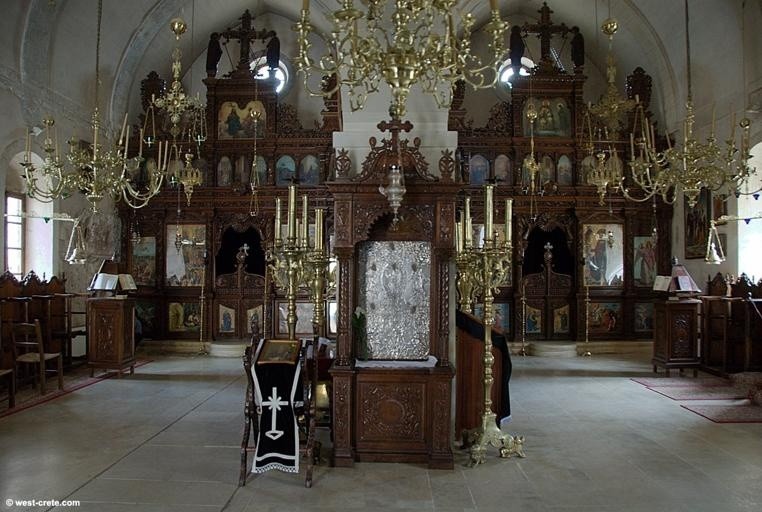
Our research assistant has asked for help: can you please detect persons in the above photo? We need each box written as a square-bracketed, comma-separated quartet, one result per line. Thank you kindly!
[557, 102, 570, 132]
[527, 104, 538, 134]
[185, 311, 200, 327]
[639, 240, 656, 285]
[250, 310, 259, 336]
[225, 106, 256, 135]
[223, 310, 231, 331]
[603, 309, 617, 332]
[494, 312, 504, 331]
[558, 309, 567, 330]
[526, 311, 537, 331]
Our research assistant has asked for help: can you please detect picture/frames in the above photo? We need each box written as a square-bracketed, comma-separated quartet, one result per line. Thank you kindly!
[714, 233, 727, 256]
[712, 194, 727, 226]
[684, 185, 711, 259]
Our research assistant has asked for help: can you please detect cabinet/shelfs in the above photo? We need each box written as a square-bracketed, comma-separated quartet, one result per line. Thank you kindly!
[649, 296, 702, 378]
[83, 296, 137, 382]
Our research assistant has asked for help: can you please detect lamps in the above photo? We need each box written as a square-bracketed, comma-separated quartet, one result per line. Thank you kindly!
[584, 121, 620, 204]
[288, 0, 514, 120]
[17, 0, 174, 217]
[614, 1, 756, 208]
[580, 1, 644, 159]
[143, 5, 213, 147]
[172, 129, 206, 208]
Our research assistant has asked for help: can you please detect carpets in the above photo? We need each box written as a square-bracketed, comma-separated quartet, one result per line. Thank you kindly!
[679, 401, 761, 425]
[644, 384, 747, 400]
[1, 358, 156, 418]
[628, 371, 730, 386]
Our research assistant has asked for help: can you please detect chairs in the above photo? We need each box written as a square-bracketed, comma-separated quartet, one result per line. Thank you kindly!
[9, 319, 66, 396]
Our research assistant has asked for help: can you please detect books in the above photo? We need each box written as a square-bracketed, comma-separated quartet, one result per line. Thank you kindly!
[93, 272, 137, 291]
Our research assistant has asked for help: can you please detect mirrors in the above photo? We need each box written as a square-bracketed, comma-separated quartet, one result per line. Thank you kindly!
[580, 222, 624, 290]
[163, 219, 207, 289]
